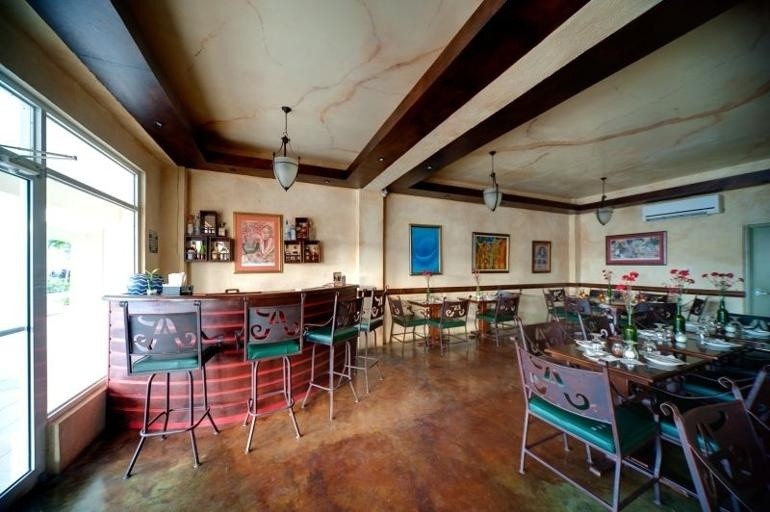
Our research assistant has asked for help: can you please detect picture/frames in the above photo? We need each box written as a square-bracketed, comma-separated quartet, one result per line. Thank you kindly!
[472, 232, 508, 273]
[531, 241, 551, 273]
[233, 211, 282, 273]
[606, 231, 666, 265]
[409, 224, 442, 276]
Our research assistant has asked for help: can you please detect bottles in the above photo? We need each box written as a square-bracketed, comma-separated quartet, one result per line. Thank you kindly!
[285, 246, 299, 262]
[333, 272, 345, 286]
[187, 239, 205, 260]
[624, 313, 637, 342]
[611, 321, 725, 360]
[305, 247, 318, 262]
[283, 220, 306, 241]
[187, 215, 226, 237]
[476, 286, 480, 294]
[212, 242, 229, 261]
[717, 300, 729, 324]
[674, 304, 685, 332]
[426, 288, 430, 301]
[607, 284, 611, 296]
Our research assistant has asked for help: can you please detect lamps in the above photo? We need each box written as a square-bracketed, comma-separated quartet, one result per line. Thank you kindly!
[483, 151, 502, 211]
[272, 106, 300, 190]
[595, 176, 615, 225]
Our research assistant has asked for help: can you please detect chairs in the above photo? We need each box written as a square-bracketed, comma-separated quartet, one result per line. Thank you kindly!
[301, 290, 364, 420]
[336, 285, 388, 394]
[243, 293, 307, 455]
[119, 301, 221, 479]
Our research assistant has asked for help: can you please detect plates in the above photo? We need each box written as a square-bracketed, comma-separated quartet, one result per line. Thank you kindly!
[643, 351, 690, 367]
[741, 329, 770, 336]
[702, 339, 743, 348]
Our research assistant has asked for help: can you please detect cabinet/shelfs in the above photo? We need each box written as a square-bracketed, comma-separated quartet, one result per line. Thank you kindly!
[284, 218, 321, 263]
[184, 235, 209, 263]
[209, 236, 233, 262]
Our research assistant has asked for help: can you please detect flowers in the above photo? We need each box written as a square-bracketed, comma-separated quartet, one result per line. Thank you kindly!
[703, 272, 745, 298]
[602, 269, 614, 285]
[421, 272, 434, 292]
[662, 268, 694, 303]
[615, 271, 646, 313]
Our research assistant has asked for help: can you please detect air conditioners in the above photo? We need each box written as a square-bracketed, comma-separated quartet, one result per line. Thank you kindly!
[641, 194, 725, 222]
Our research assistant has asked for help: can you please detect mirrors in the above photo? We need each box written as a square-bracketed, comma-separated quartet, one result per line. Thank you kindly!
[200, 211, 218, 236]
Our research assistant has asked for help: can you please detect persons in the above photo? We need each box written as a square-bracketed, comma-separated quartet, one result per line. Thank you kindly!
[242, 225, 275, 257]
